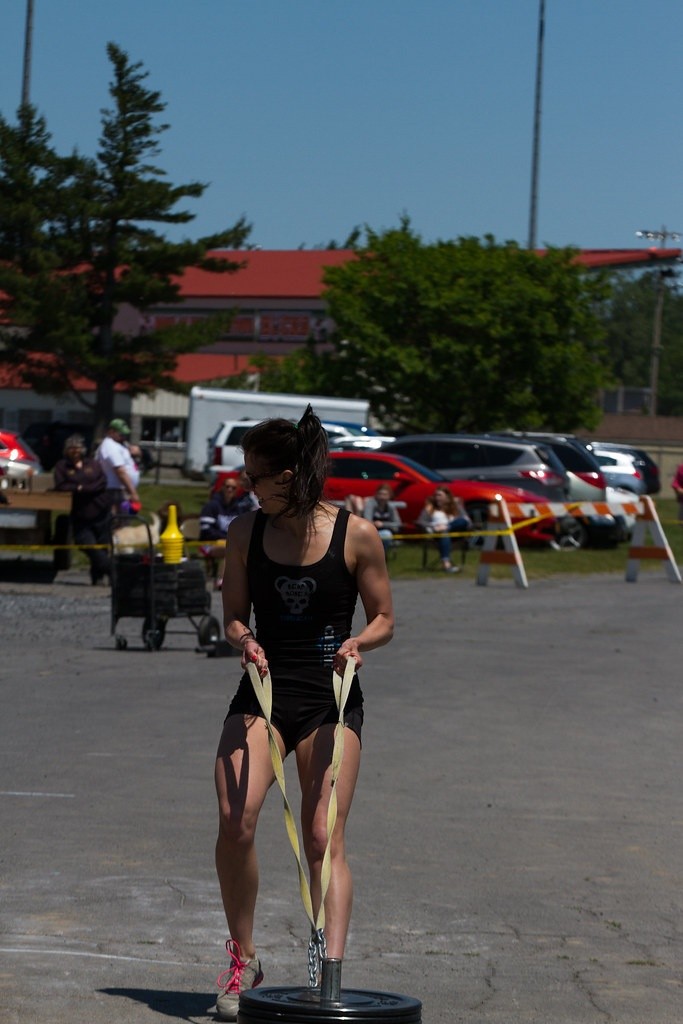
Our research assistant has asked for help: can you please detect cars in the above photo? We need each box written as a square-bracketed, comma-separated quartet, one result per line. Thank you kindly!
[185, 385, 662, 554]
[0, 429, 43, 498]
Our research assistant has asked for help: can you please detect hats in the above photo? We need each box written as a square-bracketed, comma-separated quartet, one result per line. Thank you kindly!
[109, 419, 133, 436]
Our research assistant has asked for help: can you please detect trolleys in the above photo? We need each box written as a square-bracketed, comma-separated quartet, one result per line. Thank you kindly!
[105, 509, 221, 653]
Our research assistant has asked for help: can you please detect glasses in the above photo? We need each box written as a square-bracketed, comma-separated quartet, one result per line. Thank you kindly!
[224, 484, 238, 490]
[244, 470, 277, 486]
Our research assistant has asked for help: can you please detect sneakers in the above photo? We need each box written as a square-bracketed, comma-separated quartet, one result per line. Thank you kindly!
[218, 955, 261, 1018]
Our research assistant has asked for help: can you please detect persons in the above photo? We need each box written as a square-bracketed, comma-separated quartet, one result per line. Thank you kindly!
[96, 419, 141, 528]
[212, 403, 394, 1021]
[671, 460, 683, 516]
[363, 483, 401, 563]
[415, 484, 473, 574]
[234, 467, 262, 513]
[54, 434, 115, 584]
[198, 477, 239, 590]
